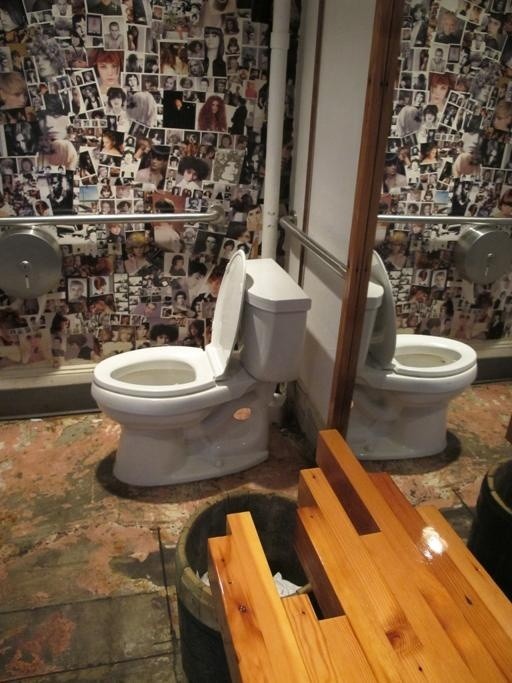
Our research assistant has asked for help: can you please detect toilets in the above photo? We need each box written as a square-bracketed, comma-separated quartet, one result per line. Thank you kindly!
[91, 250, 312, 486]
[349, 251, 479, 462]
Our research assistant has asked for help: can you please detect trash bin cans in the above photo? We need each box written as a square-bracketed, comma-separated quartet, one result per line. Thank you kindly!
[175, 489, 315, 683]
[465, 458, 512, 603]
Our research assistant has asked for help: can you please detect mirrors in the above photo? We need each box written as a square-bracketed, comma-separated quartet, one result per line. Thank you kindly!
[333, 2, 512, 610]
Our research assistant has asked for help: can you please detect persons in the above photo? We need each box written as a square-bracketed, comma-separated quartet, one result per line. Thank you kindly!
[0, 206, 286, 362]
[376, 223, 512, 342]
[0, 1, 294, 218]
[378, 1, 512, 218]
